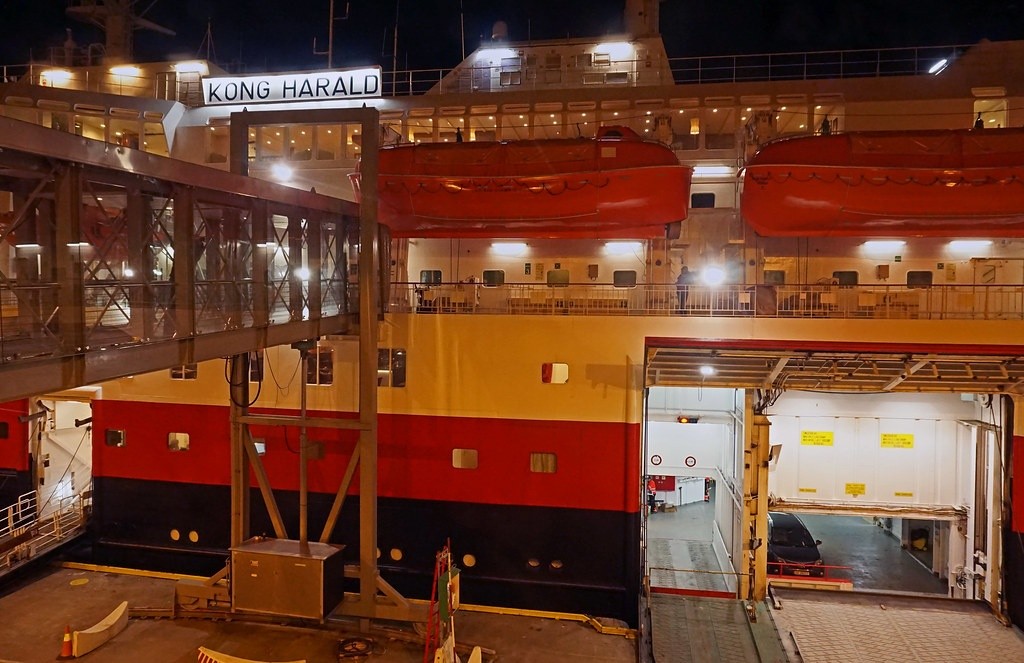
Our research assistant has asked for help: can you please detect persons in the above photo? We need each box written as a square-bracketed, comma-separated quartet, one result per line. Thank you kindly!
[648, 476, 656, 514]
[675, 266, 692, 314]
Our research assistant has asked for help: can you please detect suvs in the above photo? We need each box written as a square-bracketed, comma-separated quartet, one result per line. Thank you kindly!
[767, 511, 826, 577]
